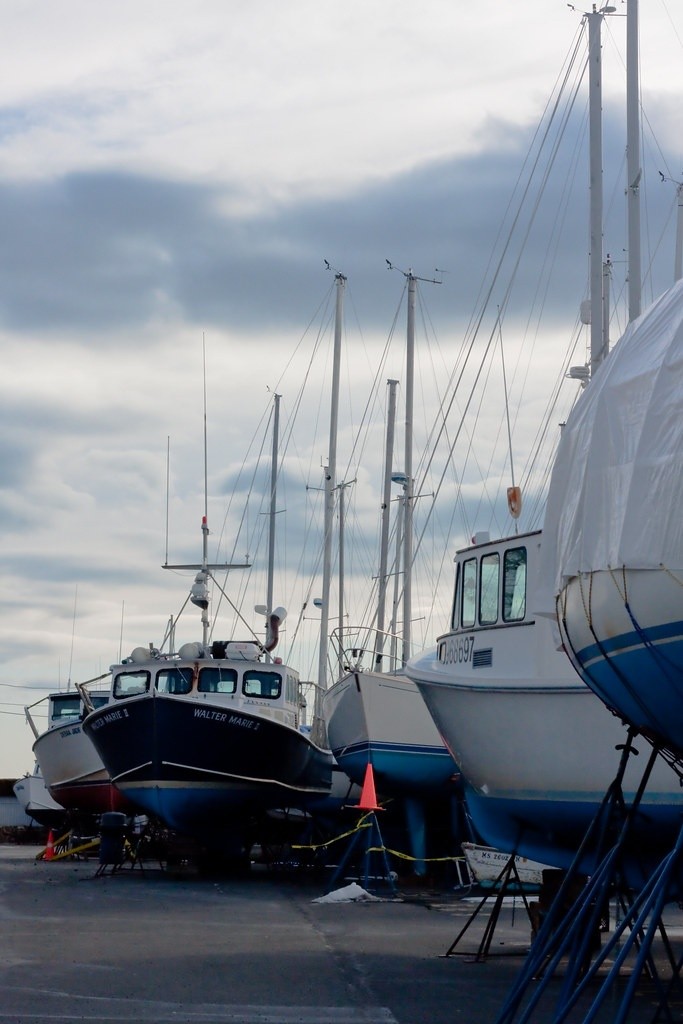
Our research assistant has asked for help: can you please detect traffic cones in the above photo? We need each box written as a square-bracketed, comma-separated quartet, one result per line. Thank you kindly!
[43, 831, 56, 859]
[353, 762, 384, 811]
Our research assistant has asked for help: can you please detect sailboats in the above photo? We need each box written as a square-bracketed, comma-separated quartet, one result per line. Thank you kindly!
[8, 1, 683, 886]
